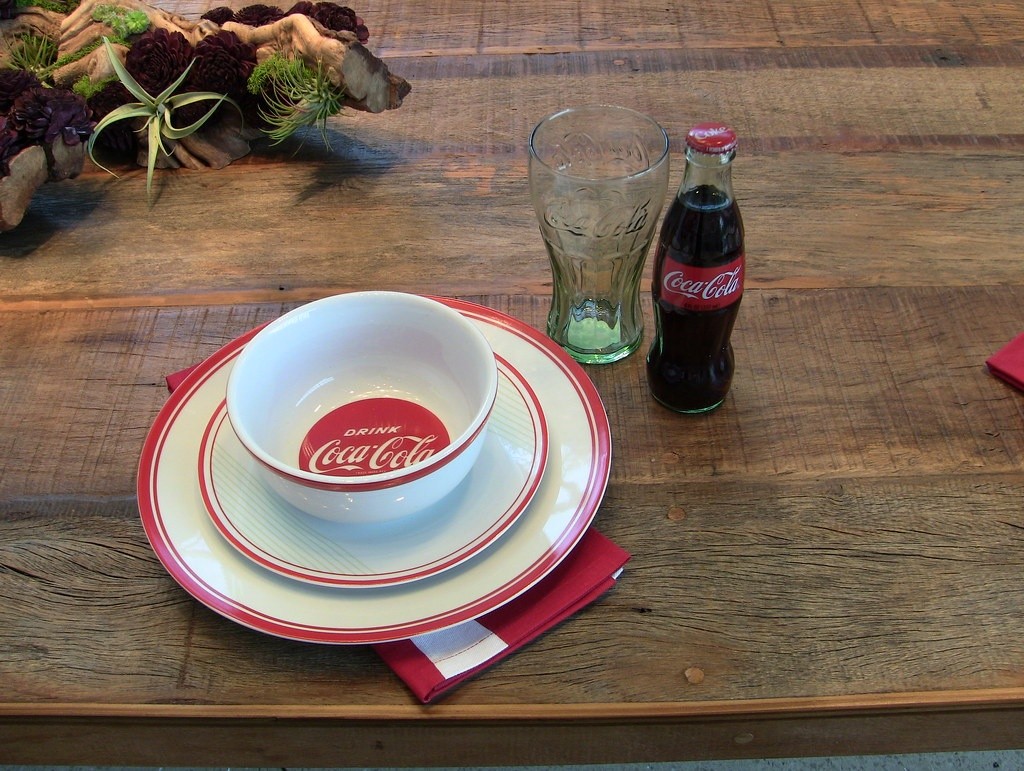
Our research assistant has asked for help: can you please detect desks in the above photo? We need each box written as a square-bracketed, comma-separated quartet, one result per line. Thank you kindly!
[1, 0, 1023, 771]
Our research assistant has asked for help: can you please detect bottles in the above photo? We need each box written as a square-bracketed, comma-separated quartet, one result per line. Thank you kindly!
[647, 124, 745, 416]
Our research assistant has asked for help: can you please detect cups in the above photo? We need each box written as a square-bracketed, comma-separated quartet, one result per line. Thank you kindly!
[530, 103, 669, 365]
[226, 290, 499, 526]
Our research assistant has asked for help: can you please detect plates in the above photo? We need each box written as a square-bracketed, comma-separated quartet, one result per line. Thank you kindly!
[199, 353, 549, 589]
[137, 295, 611, 644]
